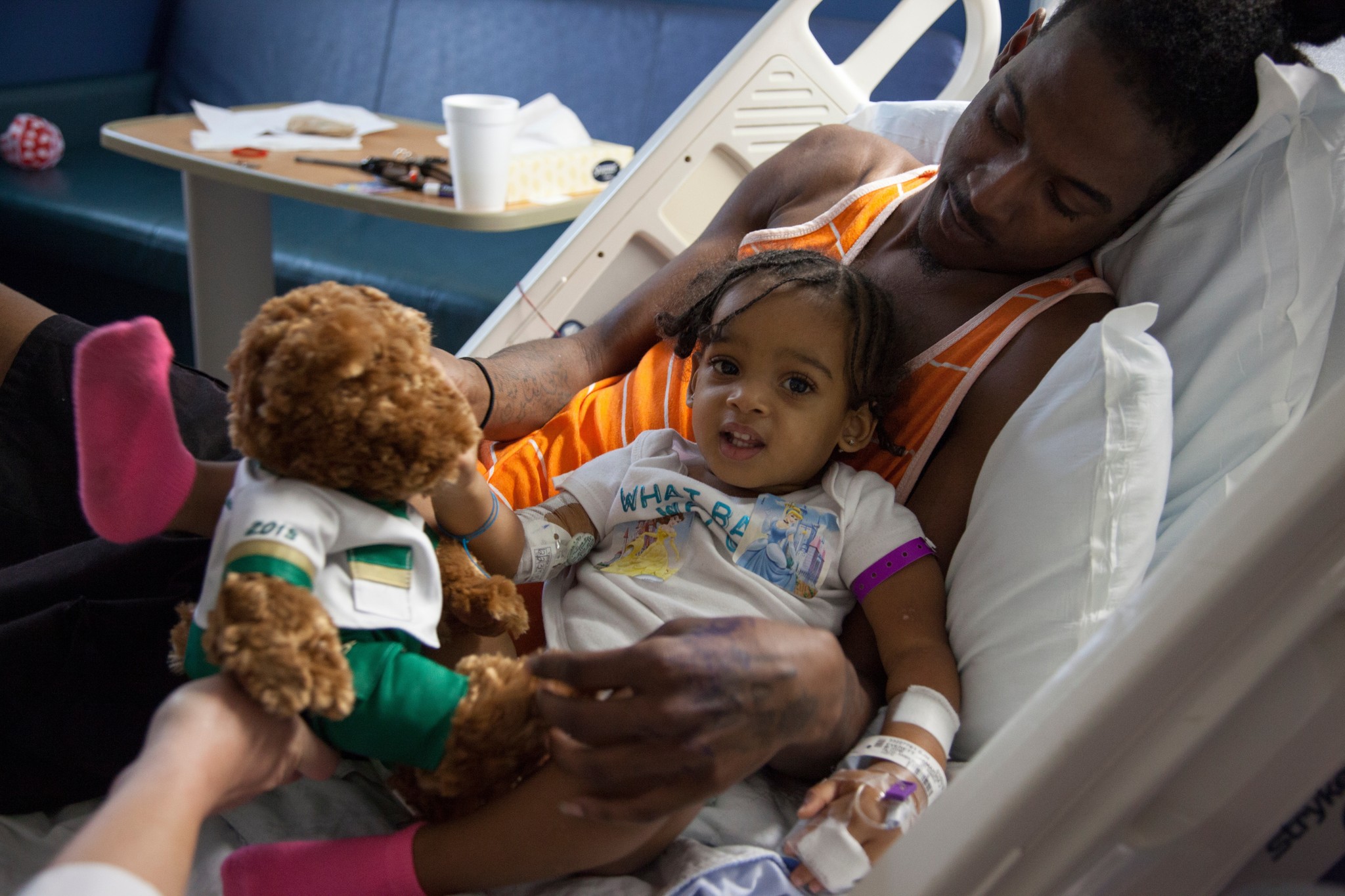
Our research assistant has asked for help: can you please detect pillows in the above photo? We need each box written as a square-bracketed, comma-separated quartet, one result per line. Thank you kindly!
[947, 301, 1172, 768]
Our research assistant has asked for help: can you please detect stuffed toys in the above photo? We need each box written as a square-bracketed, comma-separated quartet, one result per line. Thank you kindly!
[168, 277, 571, 827]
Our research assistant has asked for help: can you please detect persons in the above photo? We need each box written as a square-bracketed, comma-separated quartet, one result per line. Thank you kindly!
[0, 0, 1259, 896]
[72, 250, 966, 896]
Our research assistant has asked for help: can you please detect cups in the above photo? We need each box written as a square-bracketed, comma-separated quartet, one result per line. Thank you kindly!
[441, 87, 519, 219]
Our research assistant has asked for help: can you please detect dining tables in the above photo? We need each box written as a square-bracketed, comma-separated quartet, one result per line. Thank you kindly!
[97, 93, 638, 389]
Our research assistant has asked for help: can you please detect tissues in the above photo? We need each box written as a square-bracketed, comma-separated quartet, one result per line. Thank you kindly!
[436, 93, 635, 202]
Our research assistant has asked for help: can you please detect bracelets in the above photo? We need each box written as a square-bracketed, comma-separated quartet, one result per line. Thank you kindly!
[849, 535, 938, 604]
[461, 493, 499, 577]
[844, 731, 949, 806]
[460, 356, 495, 433]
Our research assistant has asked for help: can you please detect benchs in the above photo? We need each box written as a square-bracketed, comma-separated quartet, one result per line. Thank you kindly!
[4, 116, 582, 348]
